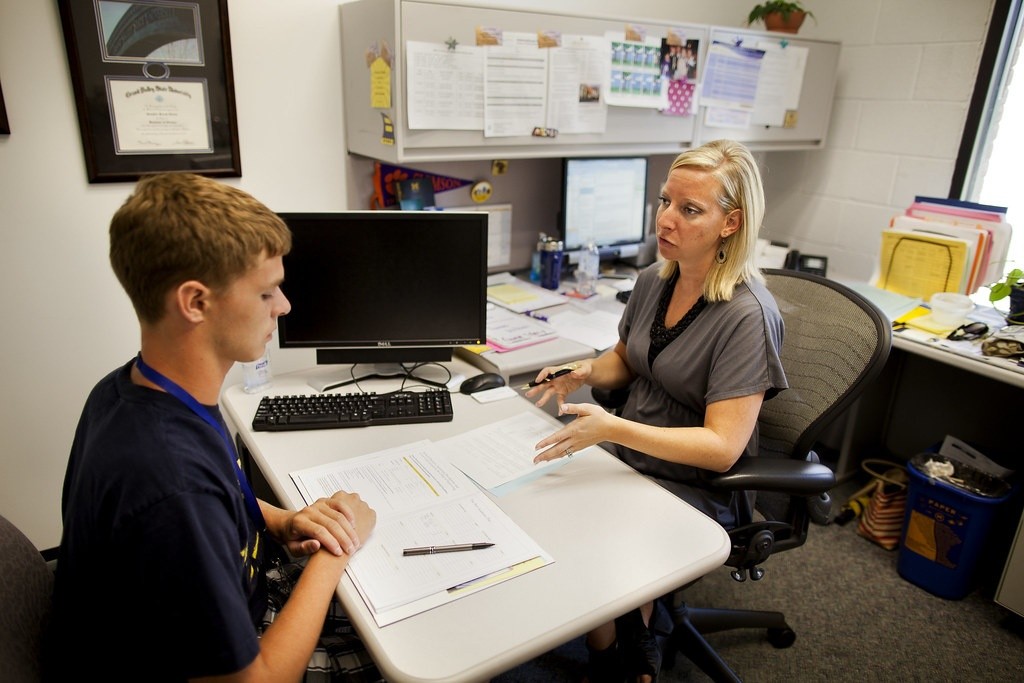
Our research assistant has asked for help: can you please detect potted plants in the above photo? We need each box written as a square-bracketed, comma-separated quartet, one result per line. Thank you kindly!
[743, 0, 815, 35]
[982, 258, 1023, 329]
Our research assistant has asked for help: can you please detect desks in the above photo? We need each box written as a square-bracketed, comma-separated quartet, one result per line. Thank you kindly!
[218, 238, 1024, 683]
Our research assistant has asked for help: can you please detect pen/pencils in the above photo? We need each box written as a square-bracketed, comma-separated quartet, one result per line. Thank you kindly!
[403, 542, 495, 556]
[520, 365, 578, 390]
[525, 310, 547, 321]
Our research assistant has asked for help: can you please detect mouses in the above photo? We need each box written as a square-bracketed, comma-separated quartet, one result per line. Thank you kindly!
[459, 373, 505, 395]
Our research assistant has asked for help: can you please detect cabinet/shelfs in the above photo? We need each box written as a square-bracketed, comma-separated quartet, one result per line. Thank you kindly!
[338, 0, 840, 177]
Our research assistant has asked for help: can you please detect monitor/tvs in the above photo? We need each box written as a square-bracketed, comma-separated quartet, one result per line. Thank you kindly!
[274, 212, 488, 392]
[559, 157, 649, 281]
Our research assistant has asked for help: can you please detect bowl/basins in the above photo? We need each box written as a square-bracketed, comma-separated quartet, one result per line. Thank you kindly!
[930, 293, 975, 325]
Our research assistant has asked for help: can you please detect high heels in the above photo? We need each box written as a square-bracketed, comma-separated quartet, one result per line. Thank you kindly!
[584, 598, 674, 683]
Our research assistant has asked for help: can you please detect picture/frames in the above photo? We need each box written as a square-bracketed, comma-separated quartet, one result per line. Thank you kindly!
[55, 0, 245, 182]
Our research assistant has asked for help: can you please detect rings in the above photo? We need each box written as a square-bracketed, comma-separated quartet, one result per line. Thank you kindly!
[566, 449, 572, 458]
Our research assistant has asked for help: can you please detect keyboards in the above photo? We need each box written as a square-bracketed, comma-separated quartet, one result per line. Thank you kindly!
[252, 388, 454, 432]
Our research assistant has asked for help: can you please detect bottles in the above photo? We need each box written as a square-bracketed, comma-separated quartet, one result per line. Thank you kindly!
[576, 236, 599, 295]
[529, 240, 564, 291]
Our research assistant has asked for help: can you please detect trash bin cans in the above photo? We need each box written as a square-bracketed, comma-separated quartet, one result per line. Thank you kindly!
[895, 452, 1004, 603]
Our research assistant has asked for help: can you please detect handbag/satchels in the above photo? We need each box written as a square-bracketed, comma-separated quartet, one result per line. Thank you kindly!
[843, 460, 910, 551]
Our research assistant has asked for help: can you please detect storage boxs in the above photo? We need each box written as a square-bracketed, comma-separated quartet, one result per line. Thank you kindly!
[899, 435, 1022, 605]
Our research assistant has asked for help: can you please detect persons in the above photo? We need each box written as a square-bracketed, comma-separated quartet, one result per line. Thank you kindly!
[660, 46, 696, 78]
[36, 172, 376, 683]
[524, 139, 789, 683]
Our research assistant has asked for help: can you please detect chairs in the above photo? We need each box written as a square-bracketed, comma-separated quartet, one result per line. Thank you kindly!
[595, 264, 890, 682]
[0, 516, 57, 683]
